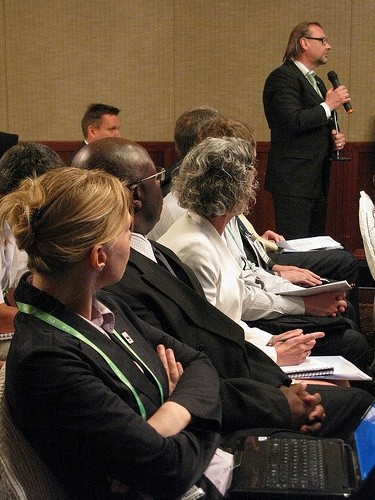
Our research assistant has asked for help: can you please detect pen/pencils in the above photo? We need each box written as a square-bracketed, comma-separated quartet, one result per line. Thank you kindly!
[277, 339, 288, 342]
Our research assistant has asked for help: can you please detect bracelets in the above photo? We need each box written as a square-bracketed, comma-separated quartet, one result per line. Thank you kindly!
[277, 271, 281, 277]
[268, 337, 273, 346]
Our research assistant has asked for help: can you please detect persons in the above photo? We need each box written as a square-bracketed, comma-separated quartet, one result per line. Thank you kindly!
[0, 103, 372, 500]
[263, 22, 351, 241]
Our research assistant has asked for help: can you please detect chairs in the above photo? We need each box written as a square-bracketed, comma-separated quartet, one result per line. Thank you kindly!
[0, 360, 71, 500]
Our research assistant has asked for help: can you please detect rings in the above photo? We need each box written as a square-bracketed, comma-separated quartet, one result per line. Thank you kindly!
[301, 275, 306, 279]
[307, 352, 310, 356]
[339, 143, 341, 147]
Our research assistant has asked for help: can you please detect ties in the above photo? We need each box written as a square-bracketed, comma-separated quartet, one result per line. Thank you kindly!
[306, 74, 323, 99]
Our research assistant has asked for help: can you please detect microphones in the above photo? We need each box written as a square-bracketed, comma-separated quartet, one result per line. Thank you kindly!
[327, 71, 353, 114]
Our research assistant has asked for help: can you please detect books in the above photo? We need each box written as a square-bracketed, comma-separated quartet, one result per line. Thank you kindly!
[280, 358, 335, 379]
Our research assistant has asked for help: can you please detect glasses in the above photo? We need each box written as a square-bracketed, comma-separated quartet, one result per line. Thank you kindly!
[135, 166, 167, 184]
[305, 36, 328, 45]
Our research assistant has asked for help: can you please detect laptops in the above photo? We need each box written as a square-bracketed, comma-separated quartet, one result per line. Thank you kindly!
[226, 399, 375, 500]
